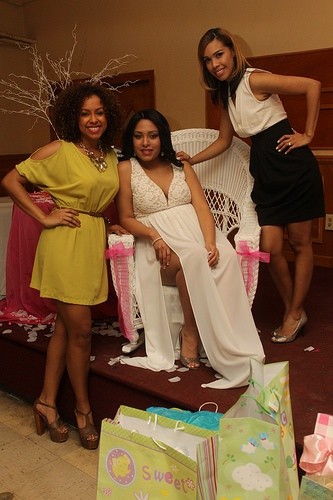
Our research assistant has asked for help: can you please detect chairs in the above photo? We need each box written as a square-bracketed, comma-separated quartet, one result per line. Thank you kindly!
[108, 128, 261, 352]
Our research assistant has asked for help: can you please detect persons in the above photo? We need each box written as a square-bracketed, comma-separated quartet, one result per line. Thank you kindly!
[176, 28, 325, 342]
[1, 84, 119, 449]
[118, 109, 266, 388]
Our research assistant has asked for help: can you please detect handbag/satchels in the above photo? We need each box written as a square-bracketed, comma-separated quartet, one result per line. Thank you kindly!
[95, 357, 333, 500]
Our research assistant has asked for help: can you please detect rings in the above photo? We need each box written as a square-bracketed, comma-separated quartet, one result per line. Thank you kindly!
[289, 142, 291, 145]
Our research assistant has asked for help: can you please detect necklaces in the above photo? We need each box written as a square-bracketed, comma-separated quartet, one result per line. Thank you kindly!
[80, 141, 107, 173]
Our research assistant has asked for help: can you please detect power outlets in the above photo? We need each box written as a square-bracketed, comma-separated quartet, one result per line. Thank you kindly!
[325, 214, 333, 230]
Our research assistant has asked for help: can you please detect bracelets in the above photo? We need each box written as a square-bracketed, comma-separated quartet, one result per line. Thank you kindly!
[152, 237, 161, 246]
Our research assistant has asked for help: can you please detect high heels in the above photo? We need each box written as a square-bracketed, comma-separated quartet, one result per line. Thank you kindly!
[74, 406, 100, 448]
[272, 311, 308, 342]
[32, 398, 69, 442]
[178, 330, 200, 369]
